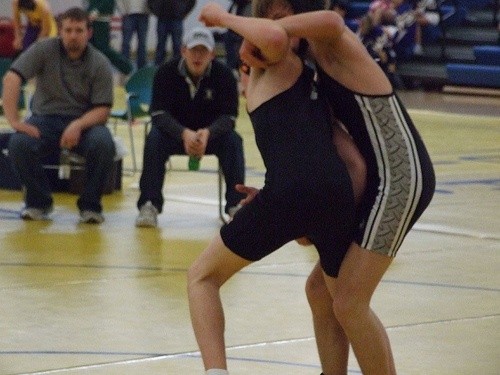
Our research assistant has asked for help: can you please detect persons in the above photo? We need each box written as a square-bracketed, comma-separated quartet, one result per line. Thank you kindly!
[114, 0, 148, 72]
[329, 0, 358, 32]
[12, 0, 58, 51]
[137, 27, 245, 229]
[147, 0, 196, 68]
[250, 0, 436, 375]
[186, 2, 366, 375]
[3, 8, 114, 223]
[353, 0, 457, 86]
[86, 0, 115, 55]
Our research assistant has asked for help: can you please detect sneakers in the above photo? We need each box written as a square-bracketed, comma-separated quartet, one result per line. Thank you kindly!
[79, 210, 106, 223]
[135, 200, 160, 229]
[20, 202, 55, 220]
[220, 206, 235, 225]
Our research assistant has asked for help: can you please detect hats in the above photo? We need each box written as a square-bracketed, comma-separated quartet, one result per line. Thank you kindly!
[182, 27, 215, 51]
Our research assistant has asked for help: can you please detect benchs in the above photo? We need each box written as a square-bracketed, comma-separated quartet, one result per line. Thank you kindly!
[349, 0, 500, 89]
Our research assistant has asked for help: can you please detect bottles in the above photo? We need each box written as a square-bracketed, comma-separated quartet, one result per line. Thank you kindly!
[189, 156, 199, 171]
[58, 142, 71, 180]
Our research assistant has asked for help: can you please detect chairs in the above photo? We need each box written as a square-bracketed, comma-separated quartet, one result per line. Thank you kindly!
[0, 15, 228, 226]
